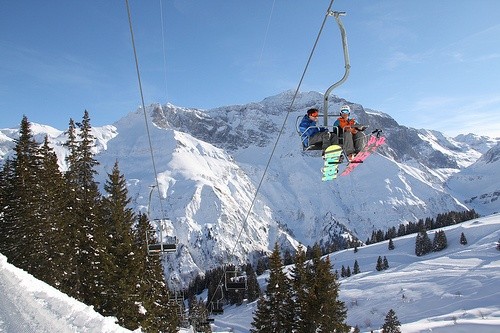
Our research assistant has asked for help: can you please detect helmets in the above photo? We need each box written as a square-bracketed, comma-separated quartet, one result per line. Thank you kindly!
[339, 105, 351, 115]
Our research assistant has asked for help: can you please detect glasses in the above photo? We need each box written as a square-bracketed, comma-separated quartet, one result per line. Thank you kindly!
[341, 110, 349, 114]
[310, 114, 318, 117]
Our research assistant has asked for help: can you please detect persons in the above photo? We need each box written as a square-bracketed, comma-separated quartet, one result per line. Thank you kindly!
[333, 106, 367, 161]
[299, 108, 344, 162]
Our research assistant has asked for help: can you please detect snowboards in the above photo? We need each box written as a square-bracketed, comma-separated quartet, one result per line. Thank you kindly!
[339, 137, 387, 176]
[321, 145, 343, 181]
